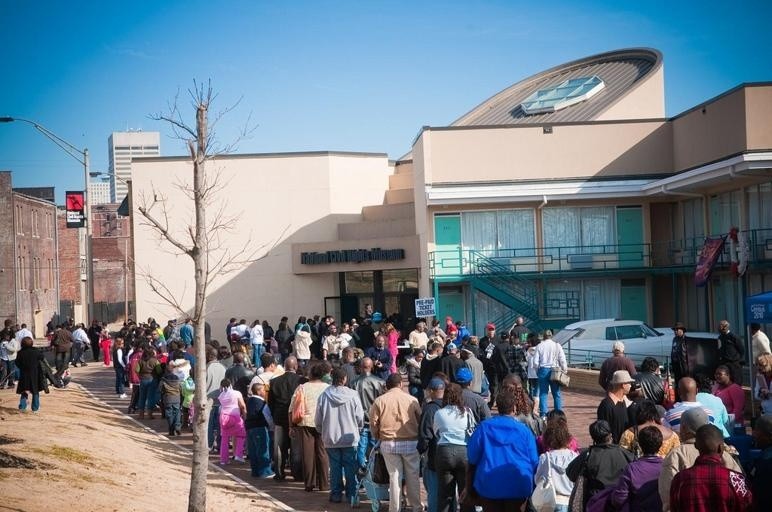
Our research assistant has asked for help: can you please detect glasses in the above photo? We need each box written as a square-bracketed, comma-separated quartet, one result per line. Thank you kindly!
[488, 329, 495, 332]
[713, 372, 727, 376]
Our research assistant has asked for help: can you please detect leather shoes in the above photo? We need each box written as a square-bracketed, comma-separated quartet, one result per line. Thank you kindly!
[305, 485, 316, 491]
[295, 477, 304, 482]
[273, 473, 287, 480]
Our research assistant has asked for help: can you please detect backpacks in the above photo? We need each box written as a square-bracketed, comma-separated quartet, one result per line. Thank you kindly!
[262, 383, 271, 402]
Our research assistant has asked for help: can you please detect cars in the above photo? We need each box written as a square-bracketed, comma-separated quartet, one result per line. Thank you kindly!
[542, 318, 724, 382]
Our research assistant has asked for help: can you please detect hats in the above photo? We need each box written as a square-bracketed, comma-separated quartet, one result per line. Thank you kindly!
[487, 324, 495, 329]
[454, 368, 473, 385]
[610, 370, 636, 386]
[612, 341, 624, 353]
[426, 378, 445, 390]
[446, 316, 453, 321]
[671, 322, 687, 334]
[501, 330, 510, 336]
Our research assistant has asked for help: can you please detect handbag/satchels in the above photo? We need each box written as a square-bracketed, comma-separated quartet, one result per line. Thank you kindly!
[629, 444, 640, 461]
[567, 475, 584, 512]
[468, 427, 478, 437]
[530, 475, 557, 512]
[52, 336, 61, 346]
[292, 393, 307, 425]
[550, 366, 571, 389]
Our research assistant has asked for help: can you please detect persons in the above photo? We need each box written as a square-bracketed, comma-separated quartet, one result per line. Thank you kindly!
[110, 303, 578, 512]
[1, 311, 112, 415]
[565, 319, 772, 512]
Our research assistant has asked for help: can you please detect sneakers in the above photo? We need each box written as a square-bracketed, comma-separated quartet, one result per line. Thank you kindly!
[234, 454, 246, 463]
[220, 459, 231, 465]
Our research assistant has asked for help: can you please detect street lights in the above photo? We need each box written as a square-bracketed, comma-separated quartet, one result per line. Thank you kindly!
[90, 170, 130, 330]
[2, 115, 97, 362]
